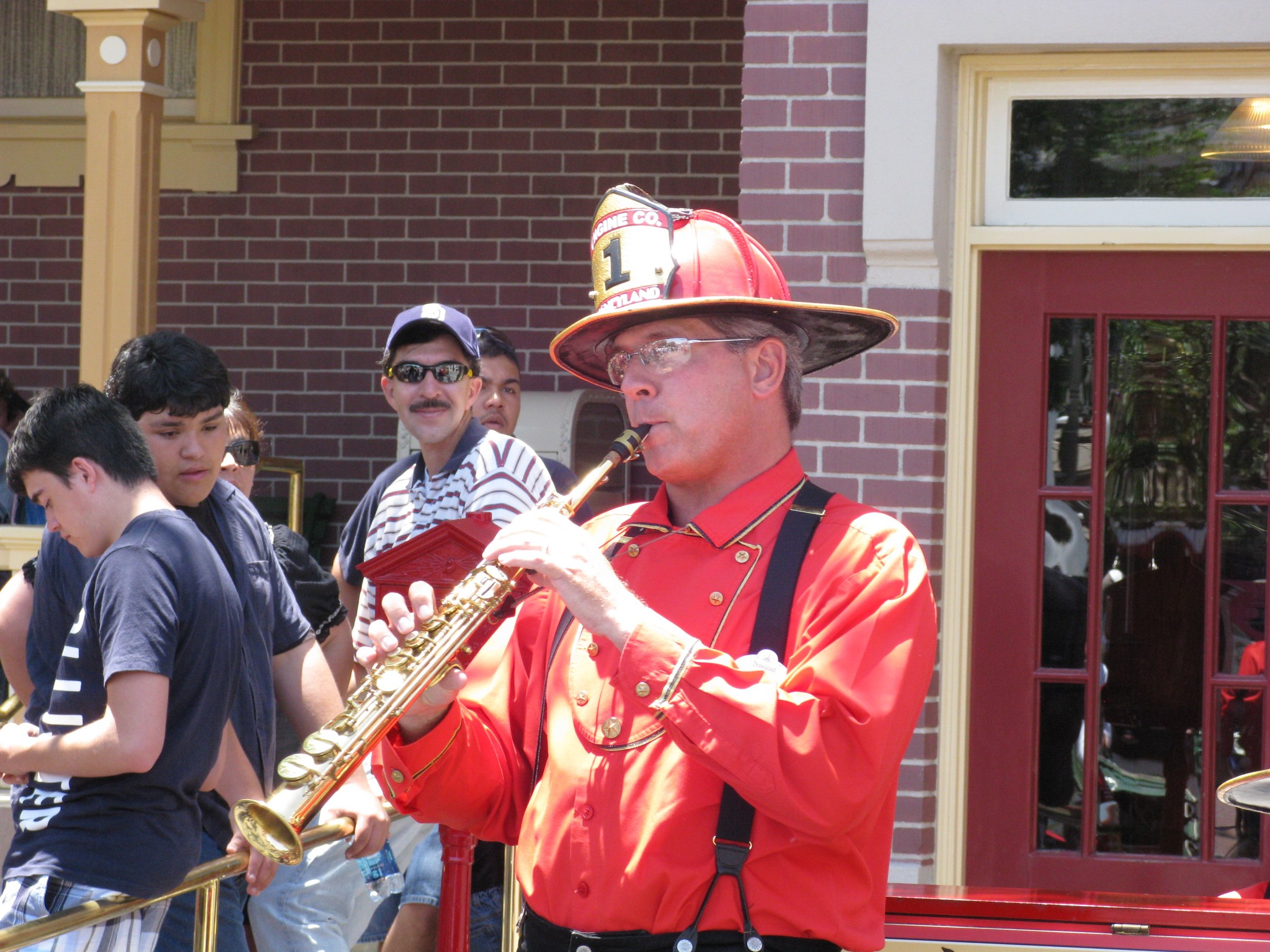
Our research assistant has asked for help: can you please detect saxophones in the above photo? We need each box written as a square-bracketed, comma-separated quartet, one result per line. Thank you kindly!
[234, 421, 655, 866]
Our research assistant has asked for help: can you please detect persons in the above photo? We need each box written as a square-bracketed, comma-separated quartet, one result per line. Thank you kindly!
[357, 184, 937, 952]
[0, 304, 591, 952]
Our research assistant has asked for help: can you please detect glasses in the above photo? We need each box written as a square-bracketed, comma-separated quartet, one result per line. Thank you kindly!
[222, 441, 260, 466]
[387, 362, 474, 383]
[606, 337, 755, 385]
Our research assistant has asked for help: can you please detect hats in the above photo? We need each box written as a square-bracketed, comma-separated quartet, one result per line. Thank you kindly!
[385, 302, 480, 357]
[549, 183, 899, 393]
